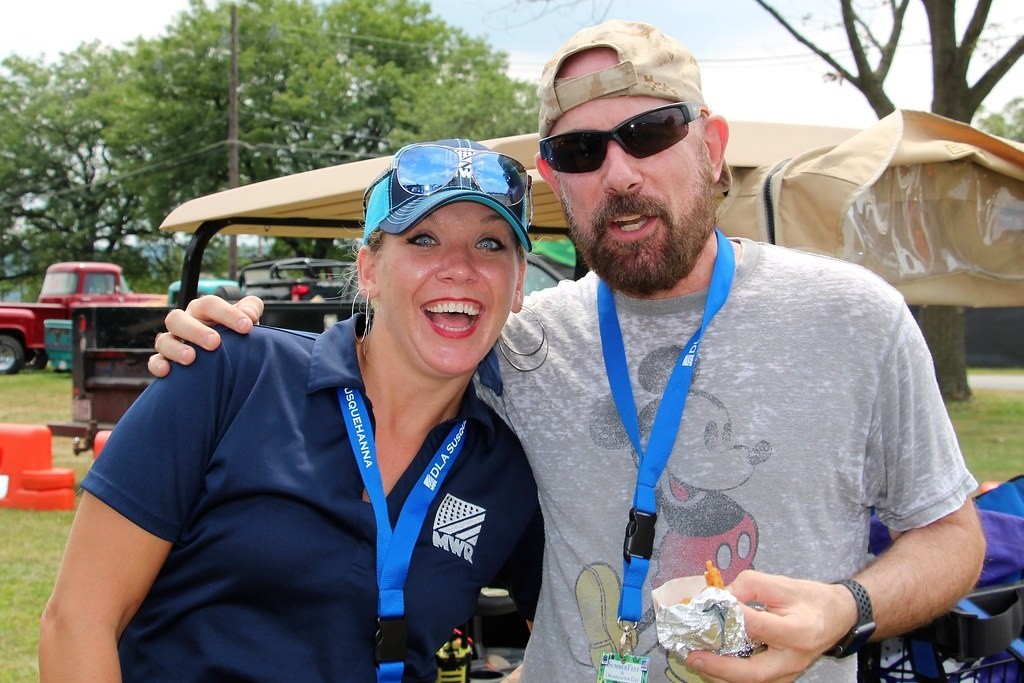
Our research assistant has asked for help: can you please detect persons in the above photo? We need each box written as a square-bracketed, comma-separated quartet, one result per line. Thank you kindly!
[147, 20, 987, 682]
[37, 136, 546, 683]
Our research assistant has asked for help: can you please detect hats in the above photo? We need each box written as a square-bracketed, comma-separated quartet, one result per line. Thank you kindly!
[362, 138, 532, 253]
[539, 20, 733, 192]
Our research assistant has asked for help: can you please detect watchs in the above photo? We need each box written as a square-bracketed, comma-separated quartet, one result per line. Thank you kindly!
[823, 579, 876, 658]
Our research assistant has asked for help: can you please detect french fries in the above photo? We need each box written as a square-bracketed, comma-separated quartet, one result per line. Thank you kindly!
[702, 560, 725, 588]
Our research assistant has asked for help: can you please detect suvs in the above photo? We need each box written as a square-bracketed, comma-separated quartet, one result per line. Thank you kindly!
[217, 256, 357, 303]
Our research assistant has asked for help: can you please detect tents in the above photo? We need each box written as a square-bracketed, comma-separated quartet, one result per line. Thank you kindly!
[156, 107, 1024, 305]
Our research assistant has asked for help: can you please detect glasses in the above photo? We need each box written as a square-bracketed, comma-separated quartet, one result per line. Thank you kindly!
[362, 143, 534, 227]
[539, 101, 703, 174]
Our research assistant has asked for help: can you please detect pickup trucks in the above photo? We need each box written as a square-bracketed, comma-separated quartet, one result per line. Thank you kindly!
[44, 280, 234, 373]
[0, 261, 169, 375]
